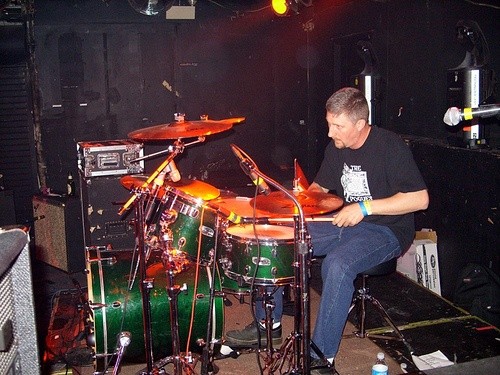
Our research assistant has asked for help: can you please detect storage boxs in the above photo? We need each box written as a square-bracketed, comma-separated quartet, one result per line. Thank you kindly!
[397, 229, 441, 297]
[76, 140, 144, 177]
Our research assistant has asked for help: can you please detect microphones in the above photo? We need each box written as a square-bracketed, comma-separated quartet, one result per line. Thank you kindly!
[169, 160, 181, 182]
[232, 145, 271, 196]
[443, 104, 500, 127]
[27, 215, 44, 223]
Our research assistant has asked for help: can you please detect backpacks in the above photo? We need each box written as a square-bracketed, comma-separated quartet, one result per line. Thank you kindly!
[454, 263, 500, 331]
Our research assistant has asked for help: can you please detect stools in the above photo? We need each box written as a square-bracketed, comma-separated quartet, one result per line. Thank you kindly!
[348, 258, 412, 354]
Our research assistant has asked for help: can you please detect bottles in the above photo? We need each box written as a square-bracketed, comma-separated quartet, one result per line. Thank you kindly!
[371, 352, 388, 375]
[67, 175, 74, 198]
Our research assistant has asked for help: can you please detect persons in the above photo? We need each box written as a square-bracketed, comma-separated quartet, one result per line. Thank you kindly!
[222, 87, 430, 375]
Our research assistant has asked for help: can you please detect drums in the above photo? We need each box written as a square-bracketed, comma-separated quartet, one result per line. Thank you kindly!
[84, 246, 225, 373]
[219, 224, 302, 287]
[143, 184, 223, 266]
[210, 195, 269, 243]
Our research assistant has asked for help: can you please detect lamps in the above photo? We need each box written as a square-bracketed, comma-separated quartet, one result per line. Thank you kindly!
[128, 0, 197, 20]
[271, 0, 312, 17]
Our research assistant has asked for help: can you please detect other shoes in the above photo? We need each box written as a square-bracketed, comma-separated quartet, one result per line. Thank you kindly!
[226, 321, 283, 346]
[310, 358, 336, 375]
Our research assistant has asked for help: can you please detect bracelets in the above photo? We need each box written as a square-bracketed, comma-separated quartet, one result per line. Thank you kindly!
[363, 200, 373, 215]
[358, 202, 367, 217]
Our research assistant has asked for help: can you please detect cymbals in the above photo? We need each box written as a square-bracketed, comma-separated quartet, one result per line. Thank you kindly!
[126, 119, 234, 140]
[248, 190, 344, 216]
[119, 174, 221, 201]
[220, 117, 246, 124]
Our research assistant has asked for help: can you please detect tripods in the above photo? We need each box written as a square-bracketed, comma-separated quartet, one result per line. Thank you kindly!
[230, 199, 339, 375]
[136, 210, 197, 375]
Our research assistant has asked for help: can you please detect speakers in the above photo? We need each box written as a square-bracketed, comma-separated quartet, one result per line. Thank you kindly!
[31, 194, 85, 274]
